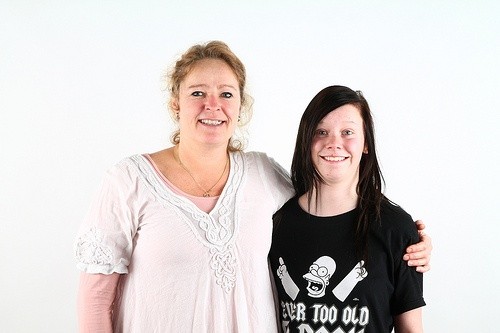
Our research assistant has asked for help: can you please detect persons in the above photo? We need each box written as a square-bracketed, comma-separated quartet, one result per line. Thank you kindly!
[267, 85, 427, 333]
[79, 40, 432, 333]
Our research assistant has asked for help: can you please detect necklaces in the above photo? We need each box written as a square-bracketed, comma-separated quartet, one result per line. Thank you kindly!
[176, 143, 230, 197]
